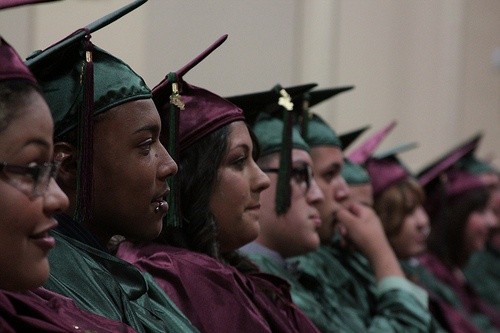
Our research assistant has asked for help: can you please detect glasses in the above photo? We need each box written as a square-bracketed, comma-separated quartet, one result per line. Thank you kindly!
[0, 160, 61, 195]
[262, 162, 314, 188]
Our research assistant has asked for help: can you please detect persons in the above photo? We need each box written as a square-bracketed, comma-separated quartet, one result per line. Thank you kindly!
[119, 35, 500, 333]
[0, 0, 132, 333]
[23, 0, 198, 333]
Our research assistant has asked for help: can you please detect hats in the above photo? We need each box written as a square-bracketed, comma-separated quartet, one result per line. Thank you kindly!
[225, 84, 317, 153]
[152, 33, 244, 148]
[0, 0, 65, 81]
[299, 84, 353, 147]
[415, 133, 500, 196]
[25, 0, 152, 143]
[341, 121, 395, 184]
[363, 142, 418, 193]
